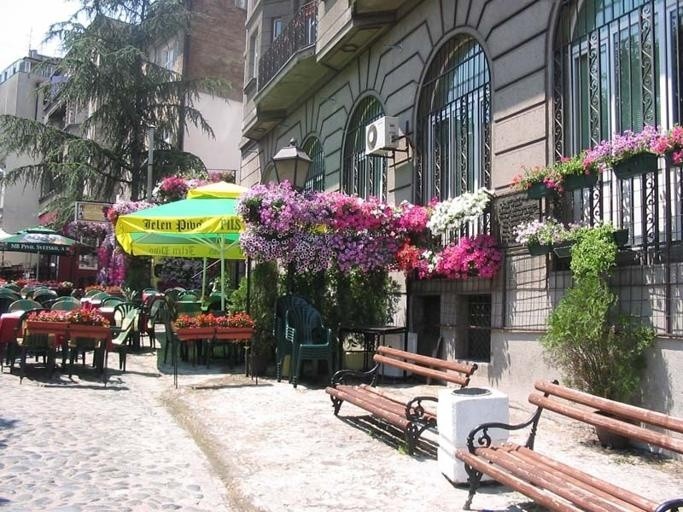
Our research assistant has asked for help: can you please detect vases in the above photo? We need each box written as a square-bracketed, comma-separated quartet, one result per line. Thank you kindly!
[550, 240, 577, 259]
[526, 243, 550, 257]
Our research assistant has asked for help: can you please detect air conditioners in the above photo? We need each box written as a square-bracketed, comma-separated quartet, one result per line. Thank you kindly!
[364, 115, 400, 156]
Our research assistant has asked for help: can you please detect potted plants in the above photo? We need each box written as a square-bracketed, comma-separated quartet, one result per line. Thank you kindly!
[539, 232, 655, 453]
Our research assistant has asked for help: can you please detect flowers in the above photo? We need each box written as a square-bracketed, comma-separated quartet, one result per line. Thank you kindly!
[510, 122, 682, 246]
[234, 174, 499, 279]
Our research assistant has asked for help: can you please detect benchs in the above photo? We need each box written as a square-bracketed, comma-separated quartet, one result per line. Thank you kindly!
[324, 344, 477, 456]
[454, 378, 682, 512]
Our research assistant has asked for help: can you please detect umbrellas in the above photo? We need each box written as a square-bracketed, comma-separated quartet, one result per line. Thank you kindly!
[0, 222, 98, 286]
[110, 179, 327, 312]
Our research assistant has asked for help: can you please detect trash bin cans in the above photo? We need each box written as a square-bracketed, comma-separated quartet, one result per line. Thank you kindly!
[437, 385, 509, 484]
